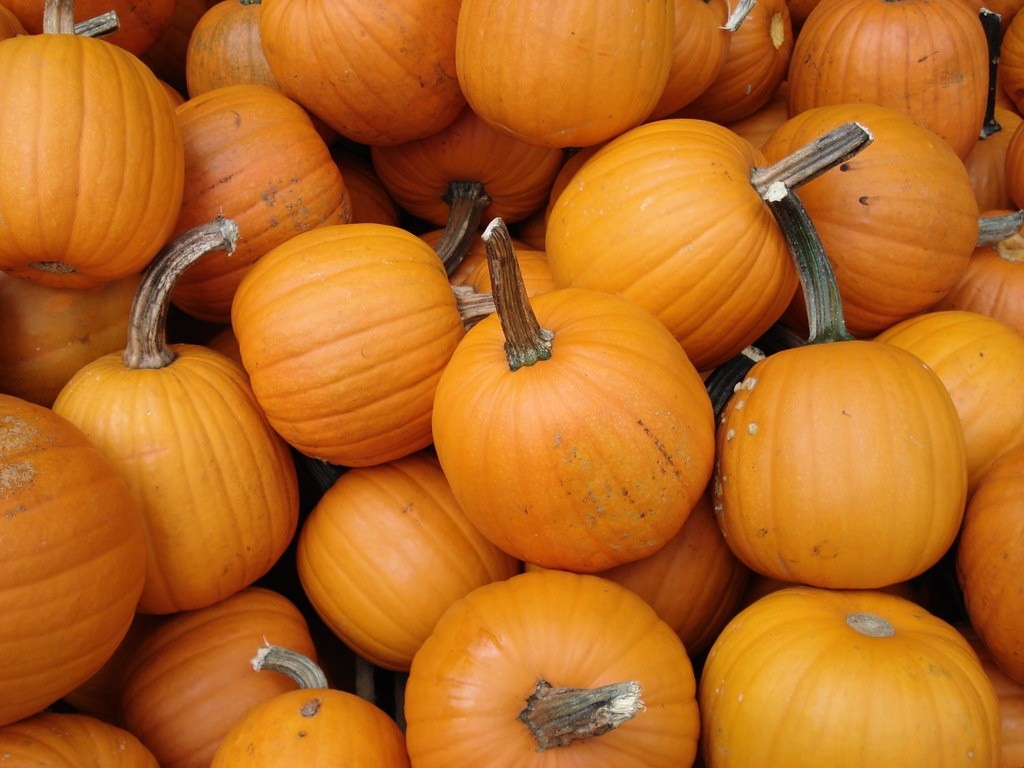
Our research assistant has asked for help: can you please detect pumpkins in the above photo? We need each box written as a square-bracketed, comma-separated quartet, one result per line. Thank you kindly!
[1, 0, 1024, 768]
